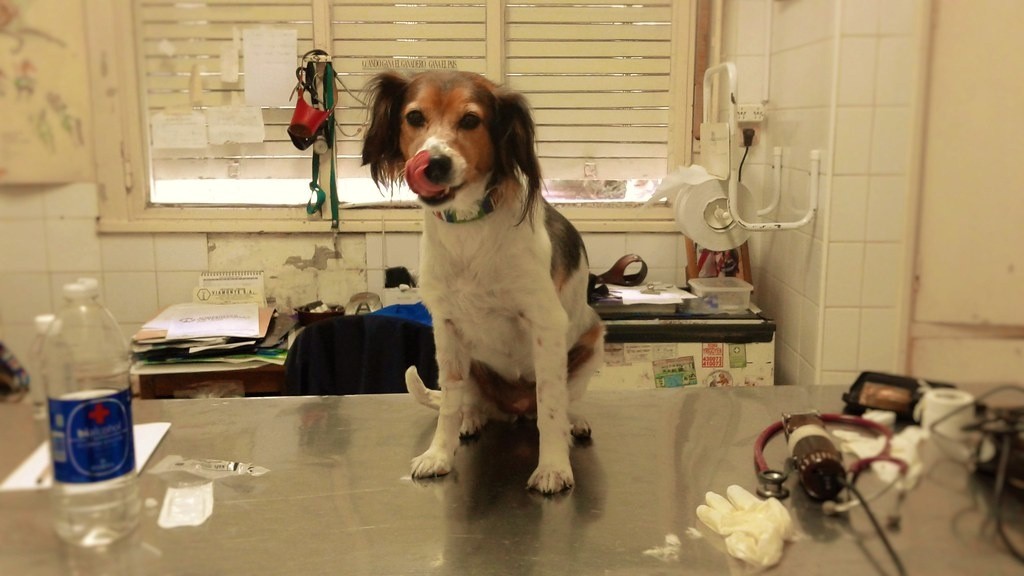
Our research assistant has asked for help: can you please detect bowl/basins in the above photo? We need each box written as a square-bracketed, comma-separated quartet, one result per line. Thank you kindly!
[688, 275, 755, 311]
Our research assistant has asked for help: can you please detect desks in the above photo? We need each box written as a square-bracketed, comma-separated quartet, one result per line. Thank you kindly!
[1, 378, 1024, 576]
[140, 365, 285, 399]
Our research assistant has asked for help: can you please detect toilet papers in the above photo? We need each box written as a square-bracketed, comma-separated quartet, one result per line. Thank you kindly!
[644, 165, 755, 251]
[914, 388, 974, 438]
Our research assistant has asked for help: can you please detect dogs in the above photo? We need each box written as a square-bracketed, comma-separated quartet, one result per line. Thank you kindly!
[360, 68, 608, 495]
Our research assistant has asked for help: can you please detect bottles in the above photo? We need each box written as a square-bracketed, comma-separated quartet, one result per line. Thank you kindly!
[25, 275, 144, 552]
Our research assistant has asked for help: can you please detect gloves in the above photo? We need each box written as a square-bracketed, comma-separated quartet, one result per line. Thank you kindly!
[697, 485, 793, 567]
[833, 427, 930, 491]
